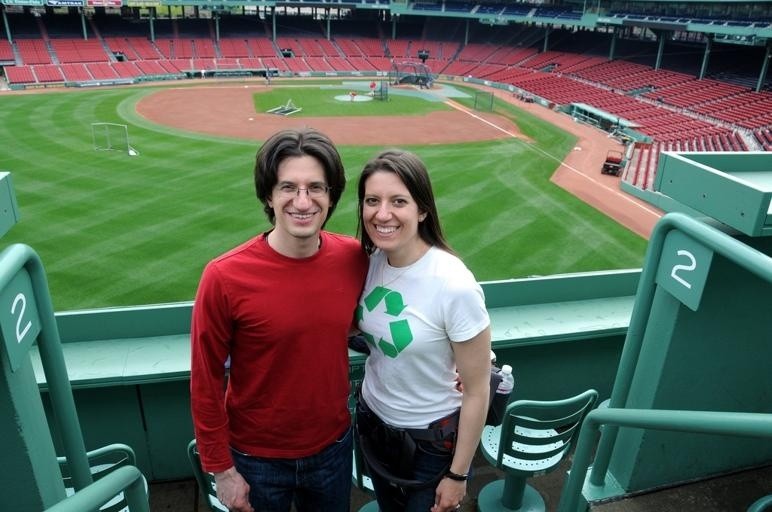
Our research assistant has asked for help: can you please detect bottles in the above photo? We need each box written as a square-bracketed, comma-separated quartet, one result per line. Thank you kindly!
[495, 364, 514, 394]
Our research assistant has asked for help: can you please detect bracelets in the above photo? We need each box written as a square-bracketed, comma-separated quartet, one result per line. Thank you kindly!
[445, 470, 469, 481]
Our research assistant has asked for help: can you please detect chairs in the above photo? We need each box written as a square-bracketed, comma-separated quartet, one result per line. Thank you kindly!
[352, 406, 381, 512]
[477, 389, 599, 512]
[56, 443, 149, 512]
[187, 438, 230, 512]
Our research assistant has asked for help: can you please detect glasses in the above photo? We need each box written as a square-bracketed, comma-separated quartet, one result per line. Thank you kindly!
[276, 182, 332, 199]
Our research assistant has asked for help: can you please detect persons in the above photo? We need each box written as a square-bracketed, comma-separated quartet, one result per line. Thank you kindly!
[349, 147, 492, 512]
[369, 80, 377, 94]
[349, 92, 357, 102]
[189, 126, 370, 512]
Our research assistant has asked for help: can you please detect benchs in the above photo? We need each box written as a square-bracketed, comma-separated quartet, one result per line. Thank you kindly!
[512, 59, 772, 192]
